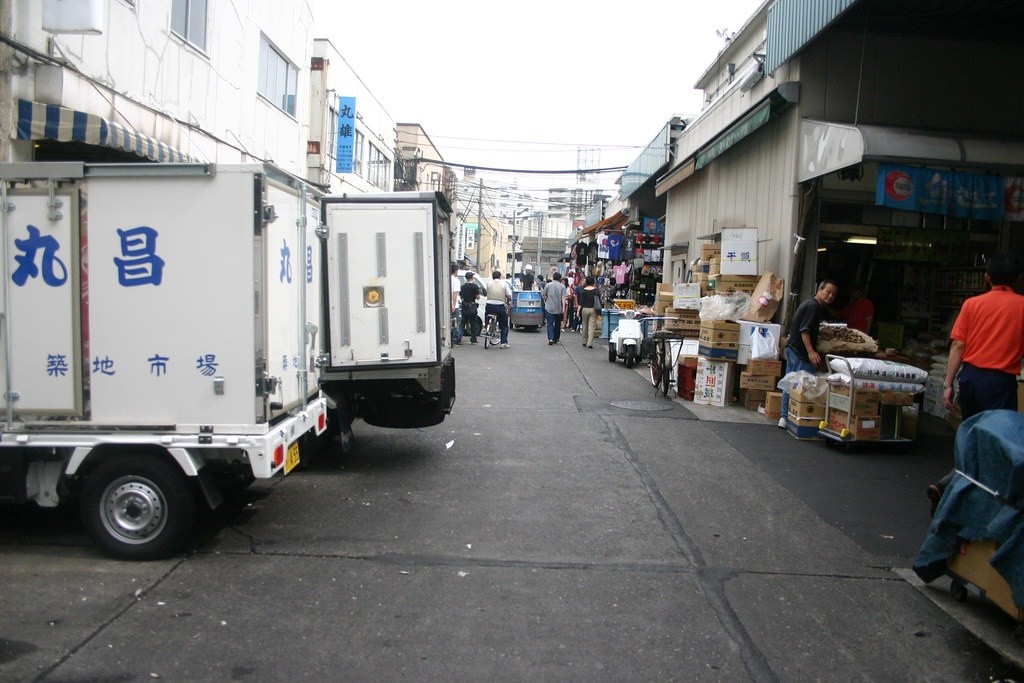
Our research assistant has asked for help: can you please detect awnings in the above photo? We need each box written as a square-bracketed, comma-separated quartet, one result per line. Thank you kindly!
[655, 81, 801, 197]
[11, 96, 202, 164]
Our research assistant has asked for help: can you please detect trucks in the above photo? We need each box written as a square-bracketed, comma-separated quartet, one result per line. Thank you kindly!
[294, 190, 457, 466]
[0, 157, 328, 560]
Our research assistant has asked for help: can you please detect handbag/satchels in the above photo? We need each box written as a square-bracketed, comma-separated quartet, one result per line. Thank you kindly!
[465, 302, 477, 317]
[594, 295, 602, 314]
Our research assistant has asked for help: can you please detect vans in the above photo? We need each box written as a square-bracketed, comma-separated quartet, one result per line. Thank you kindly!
[456, 269, 487, 334]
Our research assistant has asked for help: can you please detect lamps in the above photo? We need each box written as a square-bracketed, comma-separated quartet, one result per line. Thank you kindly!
[843, 237, 934, 248]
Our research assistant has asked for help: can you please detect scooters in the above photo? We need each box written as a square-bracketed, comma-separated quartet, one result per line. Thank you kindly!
[606, 298, 654, 368]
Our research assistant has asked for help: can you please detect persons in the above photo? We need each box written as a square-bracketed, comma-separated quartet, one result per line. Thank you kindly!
[450, 265, 461, 346]
[520, 264, 538, 291]
[927, 251, 1024, 518]
[485, 271, 512, 349]
[459, 272, 480, 345]
[543, 272, 568, 345]
[537, 270, 586, 333]
[778, 280, 837, 429]
[577, 276, 600, 348]
[832, 285, 875, 335]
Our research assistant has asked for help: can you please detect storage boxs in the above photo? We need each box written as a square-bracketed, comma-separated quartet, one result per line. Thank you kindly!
[739, 387, 766, 411]
[656, 300, 673, 316]
[923, 379, 960, 419]
[947, 535, 1024, 622]
[672, 226, 765, 310]
[698, 318, 741, 361]
[736, 344, 781, 365]
[747, 358, 781, 376]
[694, 357, 733, 406]
[829, 407, 881, 441]
[830, 384, 881, 415]
[657, 282, 673, 300]
[740, 371, 775, 391]
[788, 398, 825, 427]
[880, 391, 914, 406]
[736, 320, 781, 348]
[789, 377, 826, 404]
[664, 307, 725, 330]
[765, 391, 782, 418]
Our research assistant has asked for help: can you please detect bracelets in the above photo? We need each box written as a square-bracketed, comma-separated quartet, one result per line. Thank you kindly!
[943, 383, 953, 389]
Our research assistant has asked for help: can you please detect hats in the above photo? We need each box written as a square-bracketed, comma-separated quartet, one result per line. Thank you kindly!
[846, 280, 863, 292]
[465, 272, 475, 279]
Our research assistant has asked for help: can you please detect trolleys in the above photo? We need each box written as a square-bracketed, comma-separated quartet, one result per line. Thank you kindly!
[817, 354, 913, 455]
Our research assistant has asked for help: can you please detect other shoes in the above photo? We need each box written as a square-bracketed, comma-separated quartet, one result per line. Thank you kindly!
[589, 346, 593, 348]
[549, 339, 554, 345]
[453, 343, 462, 346]
[583, 344, 586, 347]
[471, 341, 478, 344]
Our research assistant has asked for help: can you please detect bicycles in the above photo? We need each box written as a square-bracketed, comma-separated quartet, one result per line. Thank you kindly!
[481, 303, 511, 348]
[638, 318, 686, 398]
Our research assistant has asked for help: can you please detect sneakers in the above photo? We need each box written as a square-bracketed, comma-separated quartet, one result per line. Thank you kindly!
[778, 418, 788, 429]
[481, 328, 486, 334]
[927, 482, 941, 519]
[500, 343, 512, 349]
